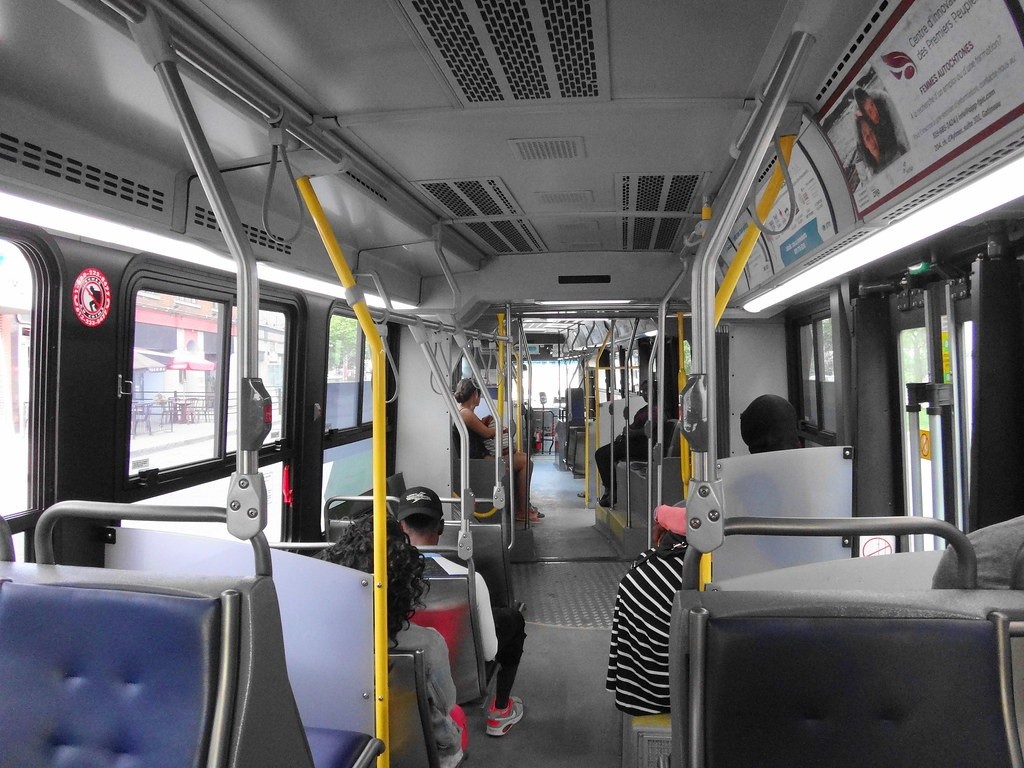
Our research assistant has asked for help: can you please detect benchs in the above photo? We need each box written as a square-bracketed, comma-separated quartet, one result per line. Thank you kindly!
[616, 457, 684, 522]
[450, 434, 512, 522]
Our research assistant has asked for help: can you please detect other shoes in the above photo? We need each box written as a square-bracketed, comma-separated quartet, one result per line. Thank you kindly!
[529, 503, 545, 518]
[599, 489, 617, 507]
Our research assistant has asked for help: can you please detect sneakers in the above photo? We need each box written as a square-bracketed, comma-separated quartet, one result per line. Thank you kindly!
[516, 511, 544, 524]
[485, 695, 524, 736]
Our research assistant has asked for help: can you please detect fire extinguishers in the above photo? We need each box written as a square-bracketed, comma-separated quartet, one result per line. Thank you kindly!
[535, 427, 542, 450]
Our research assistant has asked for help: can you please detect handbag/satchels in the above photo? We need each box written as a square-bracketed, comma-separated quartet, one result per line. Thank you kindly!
[656, 530, 688, 560]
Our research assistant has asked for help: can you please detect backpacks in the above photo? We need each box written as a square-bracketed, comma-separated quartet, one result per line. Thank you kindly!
[482, 415, 517, 456]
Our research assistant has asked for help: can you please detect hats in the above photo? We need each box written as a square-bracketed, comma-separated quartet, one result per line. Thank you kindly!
[396, 486, 444, 524]
[740, 394, 798, 454]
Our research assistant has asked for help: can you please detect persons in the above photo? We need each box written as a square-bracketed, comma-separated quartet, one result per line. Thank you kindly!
[453, 378, 546, 521]
[605, 394, 801, 718]
[321, 514, 468, 768]
[594, 380, 658, 507]
[396, 487, 525, 735]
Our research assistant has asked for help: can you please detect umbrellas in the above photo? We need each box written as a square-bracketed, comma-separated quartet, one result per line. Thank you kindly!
[133, 352, 166, 372]
[167, 348, 216, 400]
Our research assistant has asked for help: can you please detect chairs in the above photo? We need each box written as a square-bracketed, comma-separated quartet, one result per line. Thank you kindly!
[664, 581, 1024, 768]
[0, 489, 527, 768]
